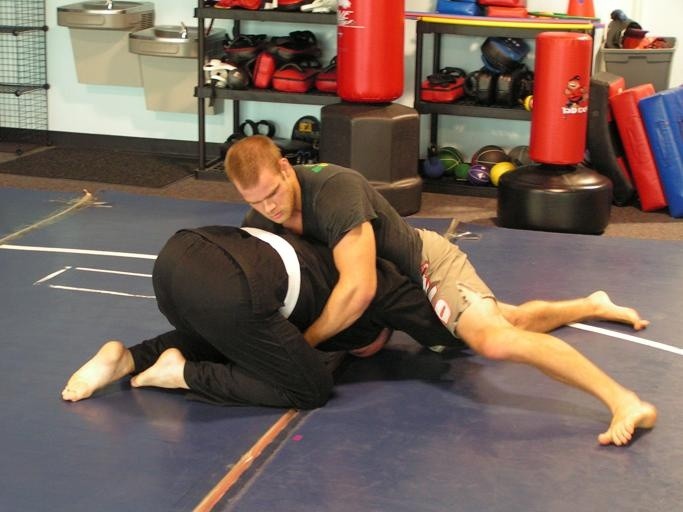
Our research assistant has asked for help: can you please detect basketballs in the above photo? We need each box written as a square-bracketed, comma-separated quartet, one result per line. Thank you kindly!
[423, 145, 530, 186]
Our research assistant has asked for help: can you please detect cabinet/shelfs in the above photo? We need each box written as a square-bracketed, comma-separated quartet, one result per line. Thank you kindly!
[193, 7, 341, 182]
[414, 18, 595, 198]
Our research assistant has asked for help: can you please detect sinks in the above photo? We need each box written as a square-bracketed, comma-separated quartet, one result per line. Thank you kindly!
[57, 2, 154, 31]
[128, 26, 225, 58]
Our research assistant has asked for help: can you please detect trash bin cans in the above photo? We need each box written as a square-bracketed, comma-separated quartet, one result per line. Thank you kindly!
[599, 37, 676, 93]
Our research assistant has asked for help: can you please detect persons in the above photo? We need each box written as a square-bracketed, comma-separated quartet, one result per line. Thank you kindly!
[61, 224, 470, 410]
[223, 134, 658, 448]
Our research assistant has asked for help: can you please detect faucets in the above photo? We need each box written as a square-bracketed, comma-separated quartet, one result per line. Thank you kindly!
[105, 0, 113, 10]
[180, 22, 188, 39]
